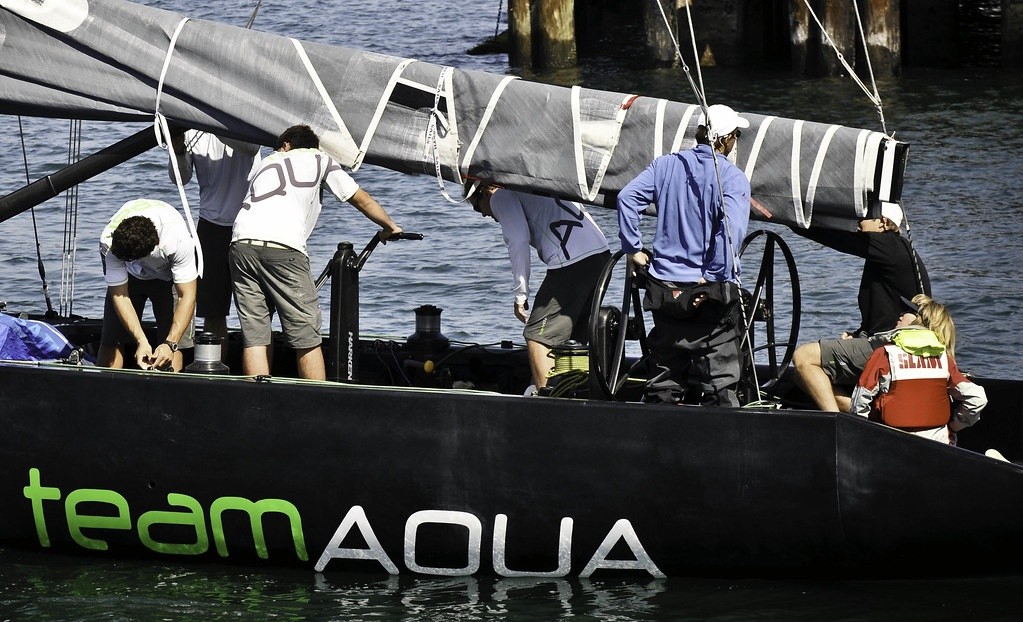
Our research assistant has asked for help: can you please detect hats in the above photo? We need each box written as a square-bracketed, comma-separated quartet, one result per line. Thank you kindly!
[899, 295, 923, 315]
[696, 103, 751, 138]
[881, 202, 903, 226]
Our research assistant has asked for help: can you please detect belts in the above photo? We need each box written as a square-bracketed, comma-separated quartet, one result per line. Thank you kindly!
[237, 238, 288, 250]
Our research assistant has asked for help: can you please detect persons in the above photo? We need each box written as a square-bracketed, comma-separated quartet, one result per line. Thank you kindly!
[160, 117, 275, 374]
[787, 201, 932, 413]
[463, 179, 613, 395]
[850, 294, 1012, 463]
[95, 199, 198, 373]
[618, 105, 751, 407]
[230, 125, 405, 382]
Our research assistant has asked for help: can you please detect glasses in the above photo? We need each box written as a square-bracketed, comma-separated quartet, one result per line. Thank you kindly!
[905, 308, 917, 317]
[473, 187, 486, 212]
[730, 130, 741, 141]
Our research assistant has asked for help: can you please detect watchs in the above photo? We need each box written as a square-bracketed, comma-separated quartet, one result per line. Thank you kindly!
[164, 340, 179, 352]
